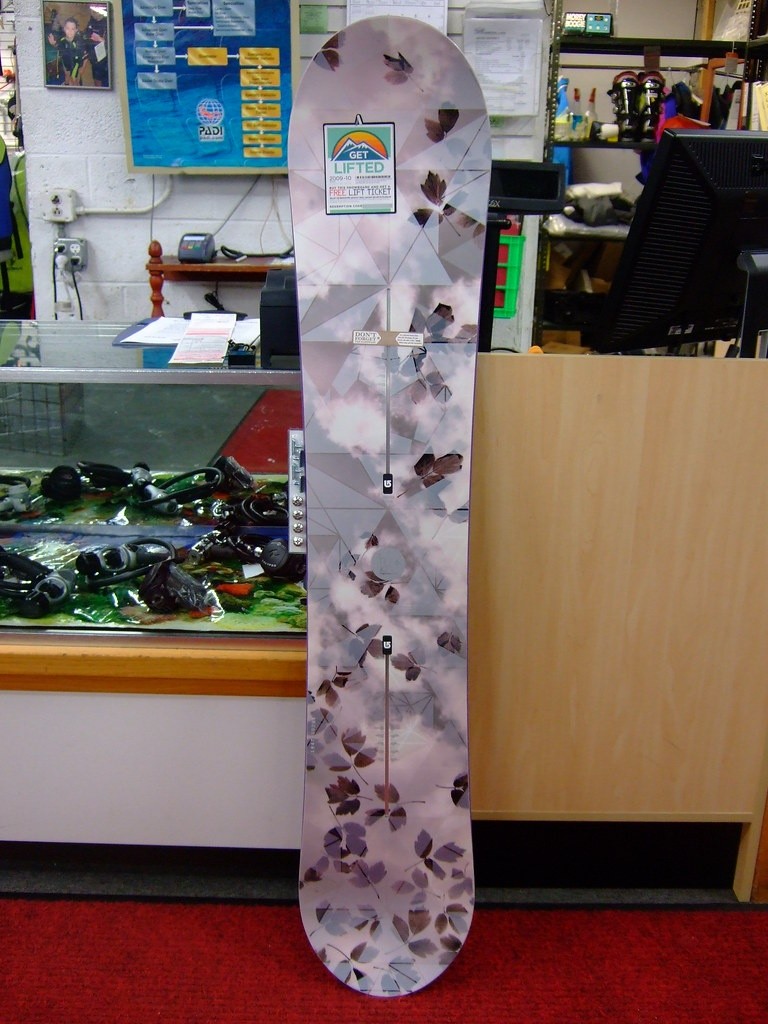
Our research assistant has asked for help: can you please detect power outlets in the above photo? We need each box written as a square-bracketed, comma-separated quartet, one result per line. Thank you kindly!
[42, 188, 77, 223]
[53, 238, 88, 272]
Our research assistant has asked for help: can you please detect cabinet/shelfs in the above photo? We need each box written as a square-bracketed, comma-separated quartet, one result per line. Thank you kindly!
[532, 0, 768, 347]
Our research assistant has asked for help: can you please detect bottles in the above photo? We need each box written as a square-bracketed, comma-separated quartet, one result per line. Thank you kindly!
[570, 88, 583, 137]
[583, 88, 598, 138]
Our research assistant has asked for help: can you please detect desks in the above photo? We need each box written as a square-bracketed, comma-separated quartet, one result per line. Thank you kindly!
[145, 241, 296, 320]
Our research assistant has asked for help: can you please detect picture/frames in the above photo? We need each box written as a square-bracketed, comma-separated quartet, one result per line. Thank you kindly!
[41, 0, 112, 90]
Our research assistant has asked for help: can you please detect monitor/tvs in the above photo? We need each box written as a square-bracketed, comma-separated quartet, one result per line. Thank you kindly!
[597, 130, 766, 359]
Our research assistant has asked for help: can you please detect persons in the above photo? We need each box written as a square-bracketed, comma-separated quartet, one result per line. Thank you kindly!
[0, 117, 35, 369]
[84, 4, 108, 87]
[48, 18, 89, 87]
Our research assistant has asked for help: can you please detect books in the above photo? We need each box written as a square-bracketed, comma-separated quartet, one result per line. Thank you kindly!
[112, 313, 260, 365]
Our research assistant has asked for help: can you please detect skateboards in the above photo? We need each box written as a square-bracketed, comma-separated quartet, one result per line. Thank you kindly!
[286, 15, 492, 995]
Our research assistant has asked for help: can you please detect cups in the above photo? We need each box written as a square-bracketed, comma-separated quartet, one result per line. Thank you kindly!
[614, 70, 638, 119]
[639, 71, 665, 116]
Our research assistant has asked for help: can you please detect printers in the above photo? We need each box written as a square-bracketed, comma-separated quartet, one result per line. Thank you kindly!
[259, 269, 302, 370]
